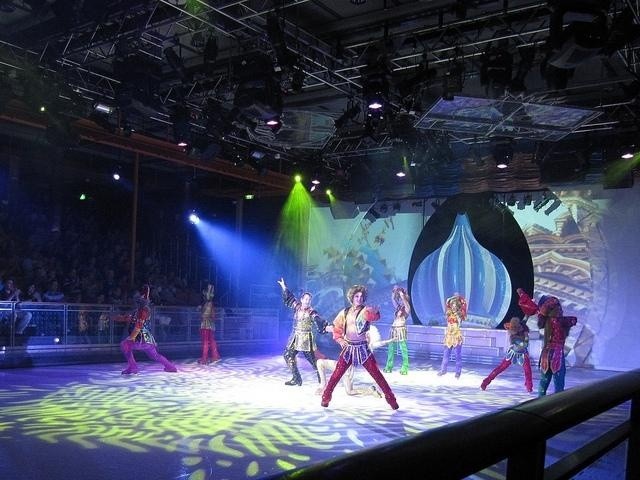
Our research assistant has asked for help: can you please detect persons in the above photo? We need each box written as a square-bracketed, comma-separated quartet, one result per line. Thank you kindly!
[535, 295, 578, 399]
[479, 314, 534, 396]
[382, 283, 411, 376]
[320, 283, 400, 411]
[109, 283, 177, 376]
[316, 324, 399, 399]
[436, 292, 468, 378]
[276, 276, 331, 386]
[191, 283, 223, 368]
[0, 172, 275, 345]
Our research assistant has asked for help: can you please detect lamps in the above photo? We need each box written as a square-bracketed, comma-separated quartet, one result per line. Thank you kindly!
[58, 0, 639, 192]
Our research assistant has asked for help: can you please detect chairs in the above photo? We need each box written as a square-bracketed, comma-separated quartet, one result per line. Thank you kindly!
[389, 400, 399, 410]
[285, 378, 302, 386]
[322, 396, 330, 406]
